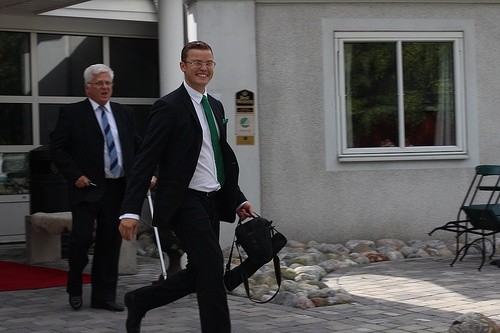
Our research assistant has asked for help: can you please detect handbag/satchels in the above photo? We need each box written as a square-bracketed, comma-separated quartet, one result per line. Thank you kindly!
[235, 215, 273, 264]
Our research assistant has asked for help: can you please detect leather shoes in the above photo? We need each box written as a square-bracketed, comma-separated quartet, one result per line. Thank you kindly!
[124, 292, 142, 333]
[91, 301, 123, 311]
[70, 294, 82, 309]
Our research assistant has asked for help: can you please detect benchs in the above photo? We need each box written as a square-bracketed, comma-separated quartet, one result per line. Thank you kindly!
[26, 212, 137, 276]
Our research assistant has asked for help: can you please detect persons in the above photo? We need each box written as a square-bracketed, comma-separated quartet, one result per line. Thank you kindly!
[119, 41, 251, 333]
[51, 63, 136, 310]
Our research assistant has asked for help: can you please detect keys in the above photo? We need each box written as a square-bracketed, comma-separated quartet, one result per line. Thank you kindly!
[88, 181, 98, 187]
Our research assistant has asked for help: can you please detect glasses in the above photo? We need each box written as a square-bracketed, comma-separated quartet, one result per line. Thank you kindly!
[183, 61, 216, 69]
[87, 81, 115, 88]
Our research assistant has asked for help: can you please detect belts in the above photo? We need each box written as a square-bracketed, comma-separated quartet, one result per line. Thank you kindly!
[188, 188, 216, 197]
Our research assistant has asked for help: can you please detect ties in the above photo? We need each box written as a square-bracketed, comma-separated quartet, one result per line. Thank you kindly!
[99, 106, 119, 176]
[201, 95, 226, 187]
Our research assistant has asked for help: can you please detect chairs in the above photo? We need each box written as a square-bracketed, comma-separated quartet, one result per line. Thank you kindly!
[427, 165, 500, 271]
